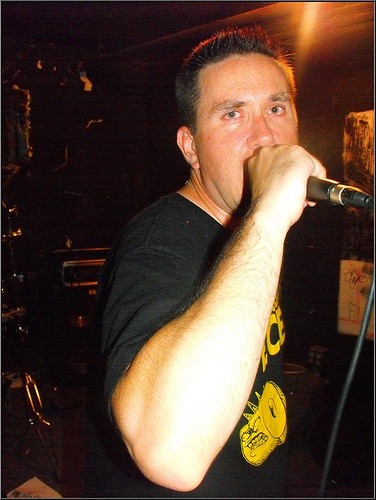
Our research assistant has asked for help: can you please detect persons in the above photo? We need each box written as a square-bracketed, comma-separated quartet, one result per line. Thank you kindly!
[85, 25, 327, 498]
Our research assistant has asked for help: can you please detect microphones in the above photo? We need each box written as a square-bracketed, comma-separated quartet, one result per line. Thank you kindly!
[305, 175, 373, 208]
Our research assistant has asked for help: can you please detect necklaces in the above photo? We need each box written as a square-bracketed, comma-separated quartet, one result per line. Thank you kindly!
[184, 181, 195, 189]
